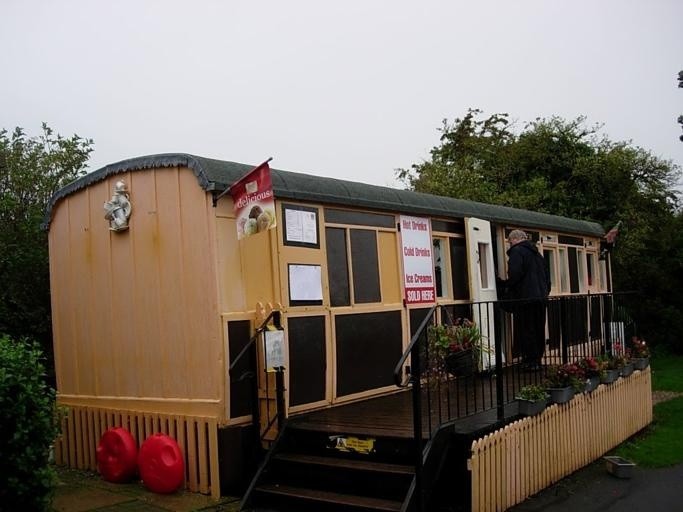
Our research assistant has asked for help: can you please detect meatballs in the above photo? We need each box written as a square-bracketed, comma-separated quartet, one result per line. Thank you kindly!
[237, 205, 275, 236]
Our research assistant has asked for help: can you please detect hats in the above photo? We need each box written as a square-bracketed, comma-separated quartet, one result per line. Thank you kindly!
[506, 230, 527, 242]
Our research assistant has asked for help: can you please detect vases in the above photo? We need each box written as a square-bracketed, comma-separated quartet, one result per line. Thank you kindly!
[439, 349, 477, 377]
[550, 387, 574, 404]
[602, 451, 637, 480]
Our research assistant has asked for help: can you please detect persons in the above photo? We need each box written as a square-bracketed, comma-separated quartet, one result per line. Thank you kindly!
[504, 229, 553, 371]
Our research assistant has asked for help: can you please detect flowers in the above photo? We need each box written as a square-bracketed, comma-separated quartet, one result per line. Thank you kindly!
[541, 334, 654, 390]
[422, 317, 497, 395]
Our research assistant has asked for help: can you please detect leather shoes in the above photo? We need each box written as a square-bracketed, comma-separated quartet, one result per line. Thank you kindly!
[513, 362, 542, 370]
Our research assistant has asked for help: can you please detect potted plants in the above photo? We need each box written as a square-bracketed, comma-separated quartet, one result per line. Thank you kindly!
[512, 383, 549, 416]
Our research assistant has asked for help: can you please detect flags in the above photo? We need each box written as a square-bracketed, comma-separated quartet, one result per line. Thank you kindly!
[228, 161, 278, 242]
